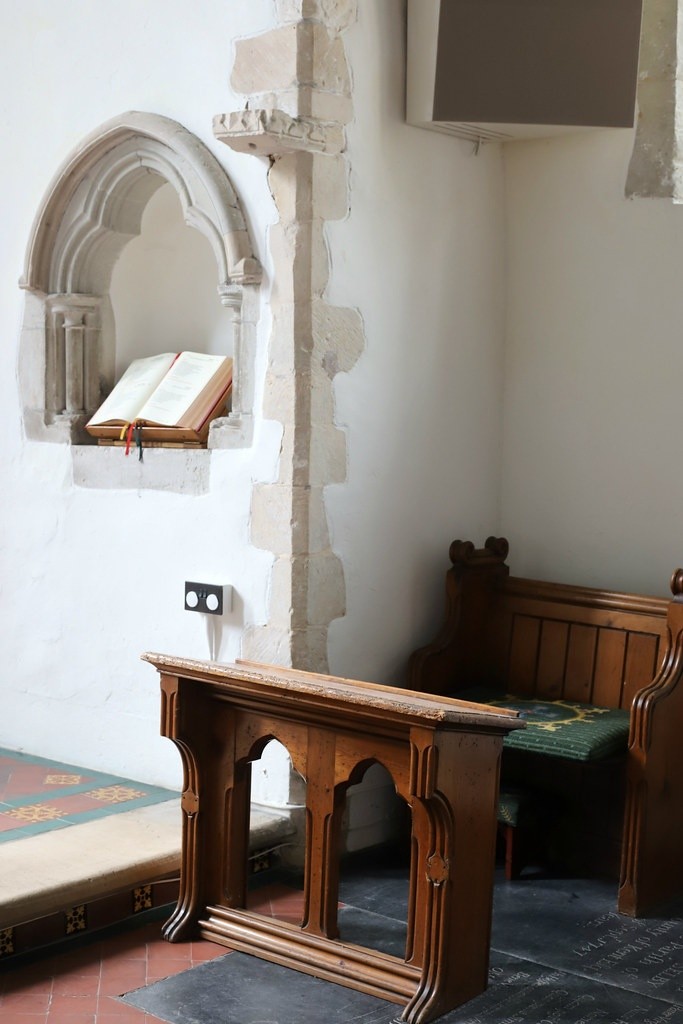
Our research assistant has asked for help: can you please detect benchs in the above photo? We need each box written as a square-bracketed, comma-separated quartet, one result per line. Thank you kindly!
[402, 536, 683, 919]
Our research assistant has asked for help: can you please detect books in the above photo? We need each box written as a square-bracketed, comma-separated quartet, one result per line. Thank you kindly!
[83, 351, 233, 432]
